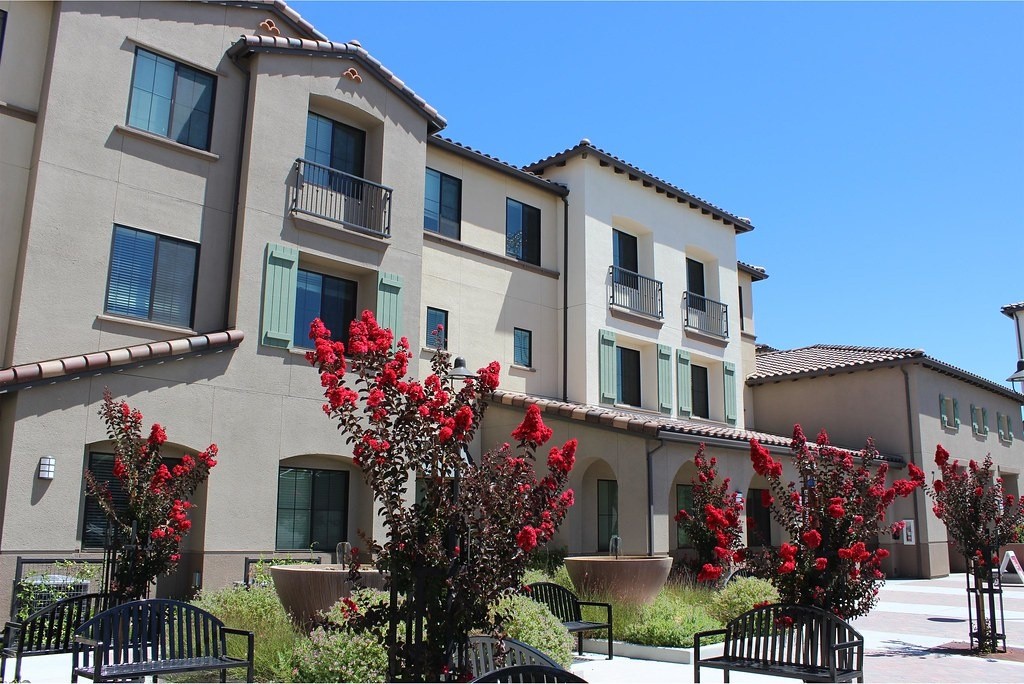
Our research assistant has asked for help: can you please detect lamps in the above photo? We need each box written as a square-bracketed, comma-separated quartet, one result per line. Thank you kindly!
[735, 490, 743, 508]
[39, 456, 55, 479]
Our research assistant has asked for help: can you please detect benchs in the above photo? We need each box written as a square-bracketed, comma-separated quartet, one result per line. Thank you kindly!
[694, 603, 864, 684]
[516, 581, 613, 660]
[0, 593, 163, 683]
[72, 599, 254, 684]
[444, 636, 590, 683]
